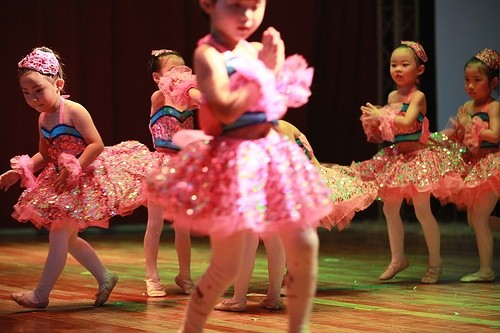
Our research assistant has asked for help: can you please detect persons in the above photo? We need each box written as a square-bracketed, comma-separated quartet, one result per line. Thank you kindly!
[360, 41, 468, 284]
[453, 48, 500, 282]
[141, 0, 334, 333]
[0, 46, 119, 309]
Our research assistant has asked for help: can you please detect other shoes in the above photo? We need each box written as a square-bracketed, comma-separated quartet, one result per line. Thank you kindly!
[175, 274, 195, 296]
[379, 257, 409, 280]
[146, 274, 166, 297]
[459, 272, 496, 283]
[95, 272, 119, 307]
[214, 301, 246, 311]
[421, 257, 443, 284]
[10, 292, 49, 309]
[256, 298, 281, 309]
